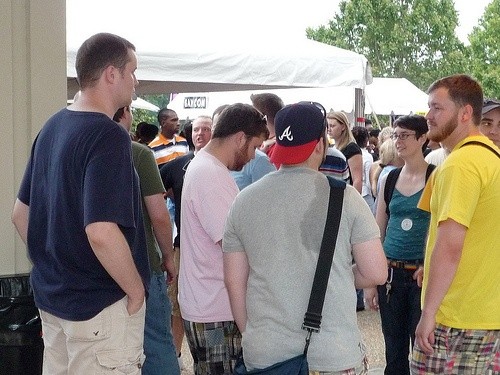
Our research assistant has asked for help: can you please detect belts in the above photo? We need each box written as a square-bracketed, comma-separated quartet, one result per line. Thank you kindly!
[387, 259, 420, 269]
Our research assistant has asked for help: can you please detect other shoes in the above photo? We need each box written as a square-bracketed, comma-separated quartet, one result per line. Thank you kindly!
[177, 357, 183, 367]
[356, 306, 364, 312]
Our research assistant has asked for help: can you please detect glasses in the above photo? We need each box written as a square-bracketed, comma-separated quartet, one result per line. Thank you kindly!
[390, 132, 416, 140]
[300, 101, 327, 120]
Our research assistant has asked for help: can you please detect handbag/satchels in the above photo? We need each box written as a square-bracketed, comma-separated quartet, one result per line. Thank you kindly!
[232, 347, 309, 375]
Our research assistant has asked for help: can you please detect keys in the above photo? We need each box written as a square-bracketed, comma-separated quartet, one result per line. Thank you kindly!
[386, 282, 392, 295]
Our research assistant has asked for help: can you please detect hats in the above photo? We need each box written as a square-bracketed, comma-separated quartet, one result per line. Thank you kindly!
[269, 102, 324, 164]
[135, 122, 158, 143]
[481, 100, 500, 114]
[179, 124, 192, 144]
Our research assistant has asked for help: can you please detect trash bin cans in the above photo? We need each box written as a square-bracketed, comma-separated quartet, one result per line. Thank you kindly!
[0, 273, 43, 375]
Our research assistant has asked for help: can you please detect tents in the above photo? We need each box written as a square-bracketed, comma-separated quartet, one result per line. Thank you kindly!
[67, 30, 430, 126]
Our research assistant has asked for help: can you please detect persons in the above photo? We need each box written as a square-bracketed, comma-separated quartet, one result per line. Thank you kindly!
[113, 92, 500, 375]
[13, 33, 147, 375]
[408, 74, 500, 375]
